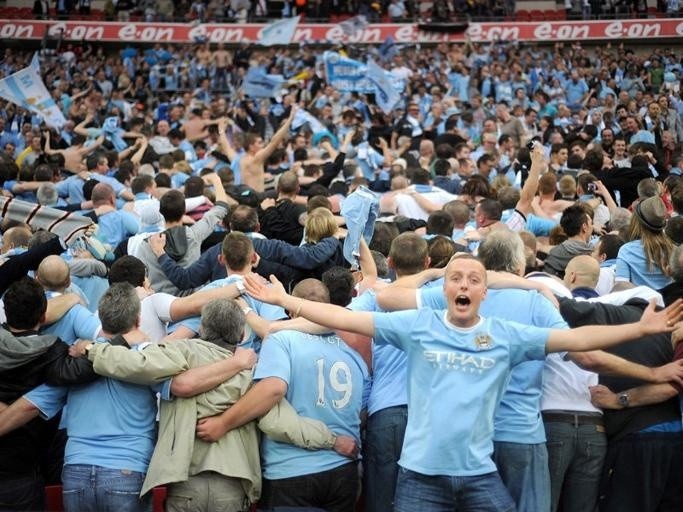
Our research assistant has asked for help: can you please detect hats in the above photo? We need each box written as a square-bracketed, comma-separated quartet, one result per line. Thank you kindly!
[632, 195, 668, 232]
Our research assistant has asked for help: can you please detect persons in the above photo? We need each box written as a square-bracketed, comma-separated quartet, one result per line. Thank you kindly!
[0, 0, 683, 511]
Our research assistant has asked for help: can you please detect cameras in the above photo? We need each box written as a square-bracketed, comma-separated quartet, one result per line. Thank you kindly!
[588, 182, 597, 192]
[529, 140, 541, 151]
